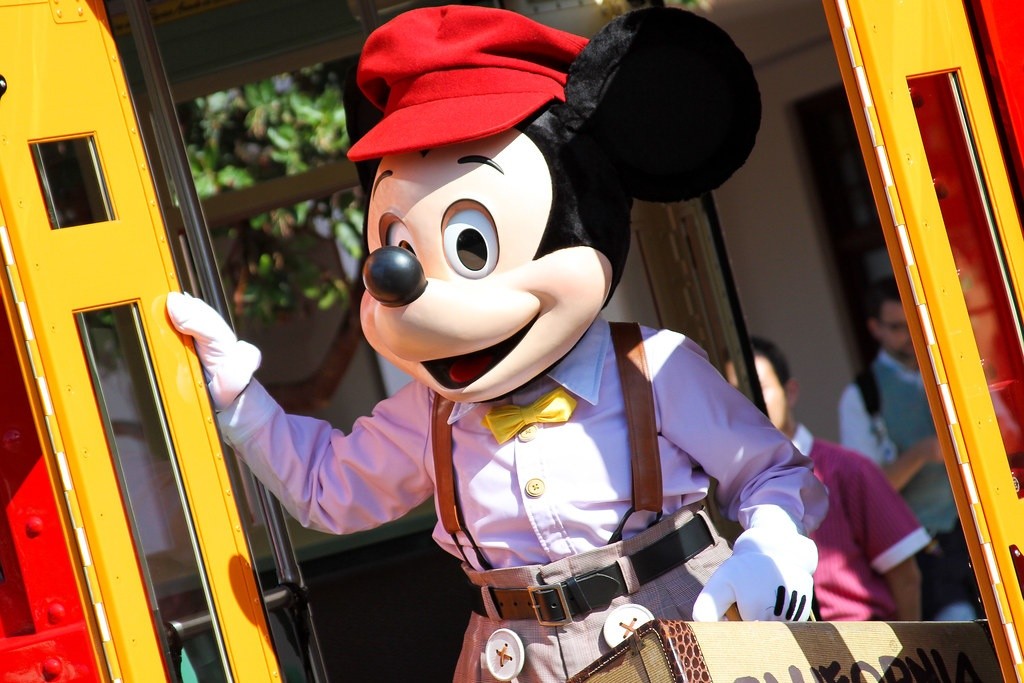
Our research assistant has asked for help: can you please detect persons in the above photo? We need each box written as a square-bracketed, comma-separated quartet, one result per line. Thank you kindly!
[830, 274, 985, 621]
[711, 331, 934, 621]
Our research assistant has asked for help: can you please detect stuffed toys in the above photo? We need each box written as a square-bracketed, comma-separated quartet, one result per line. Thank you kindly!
[166, 9, 830, 683]
